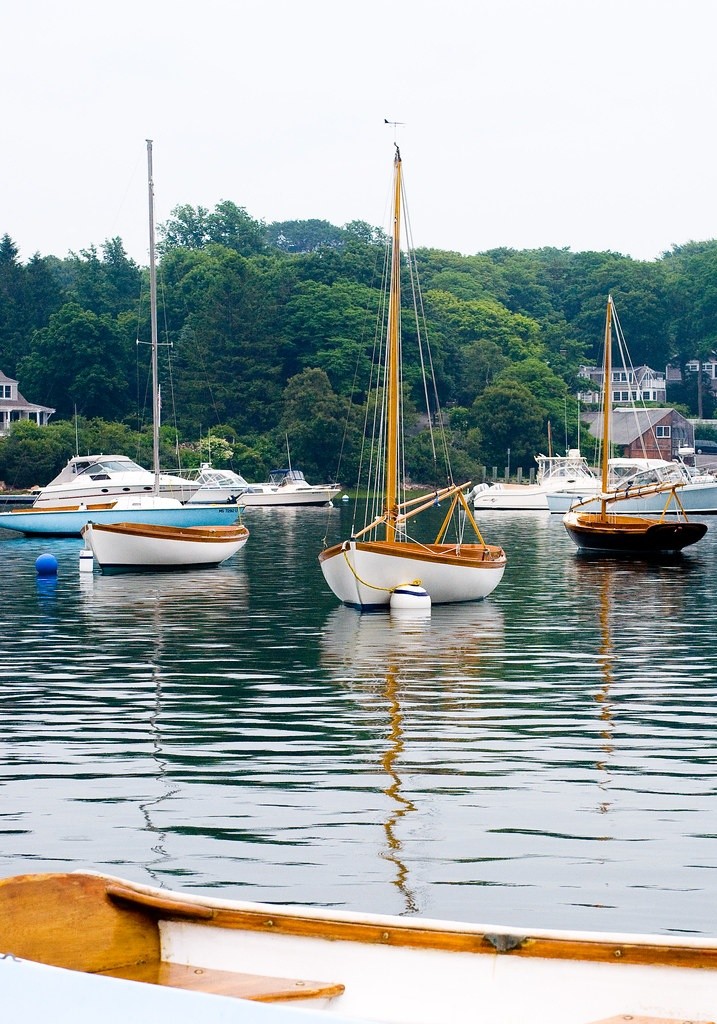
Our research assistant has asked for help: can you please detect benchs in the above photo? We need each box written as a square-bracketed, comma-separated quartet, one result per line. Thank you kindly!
[91, 958, 346, 1003]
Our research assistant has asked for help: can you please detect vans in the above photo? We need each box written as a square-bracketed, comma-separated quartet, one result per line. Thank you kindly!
[695, 440, 717, 455]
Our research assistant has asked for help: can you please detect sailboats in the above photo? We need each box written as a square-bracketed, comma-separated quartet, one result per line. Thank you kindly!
[562, 294, 709, 552]
[76, 136, 252, 576]
[318, 142, 507, 609]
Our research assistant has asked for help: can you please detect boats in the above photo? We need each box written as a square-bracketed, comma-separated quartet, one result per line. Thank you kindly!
[31, 452, 203, 509]
[546, 457, 717, 515]
[473, 455, 616, 512]
[0, 487, 249, 536]
[180, 467, 250, 504]
[225, 468, 342, 506]
